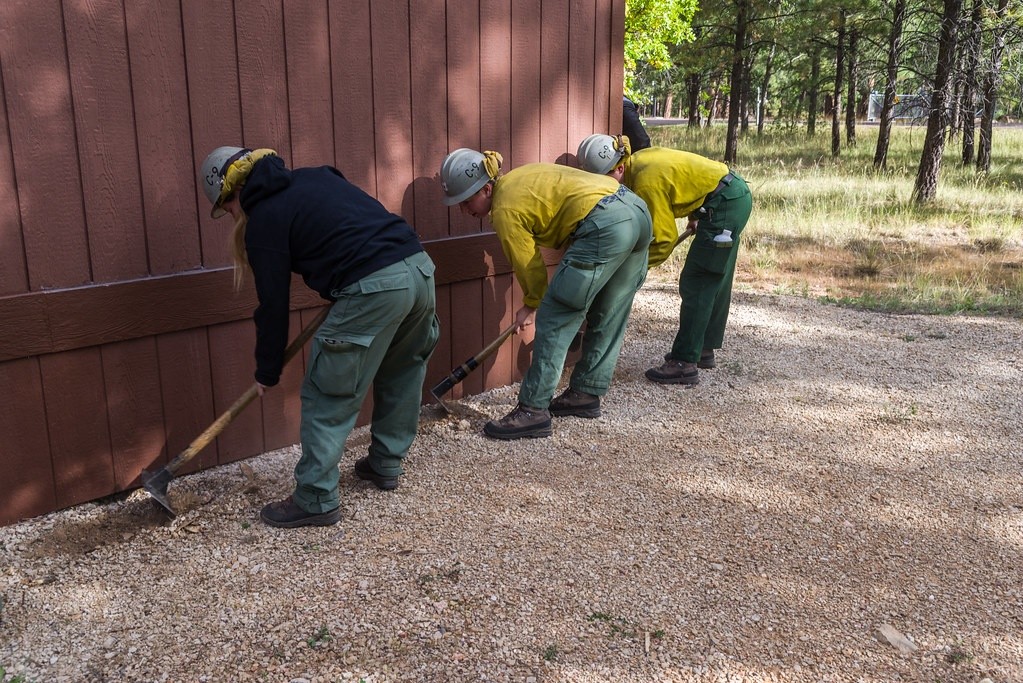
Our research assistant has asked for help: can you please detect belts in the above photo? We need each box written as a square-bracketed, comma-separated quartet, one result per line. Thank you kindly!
[703, 173, 734, 203]
[598, 184, 627, 205]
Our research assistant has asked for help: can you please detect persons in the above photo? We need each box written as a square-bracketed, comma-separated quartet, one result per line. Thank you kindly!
[623, 94, 651, 155]
[577, 134, 753, 385]
[200, 145, 441, 527]
[440, 147, 656, 440]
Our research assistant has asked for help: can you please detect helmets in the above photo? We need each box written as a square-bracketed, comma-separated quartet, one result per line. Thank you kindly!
[576, 133, 621, 175]
[200, 146, 253, 219]
[440, 148, 491, 206]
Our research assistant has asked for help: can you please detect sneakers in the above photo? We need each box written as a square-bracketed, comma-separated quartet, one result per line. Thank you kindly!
[260, 495, 342, 527]
[356, 456, 398, 489]
[665, 348, 716, 367]
[645, 359, 699, 384]
[550, 385, 601, 418]
[484, 403, 553, 439]
